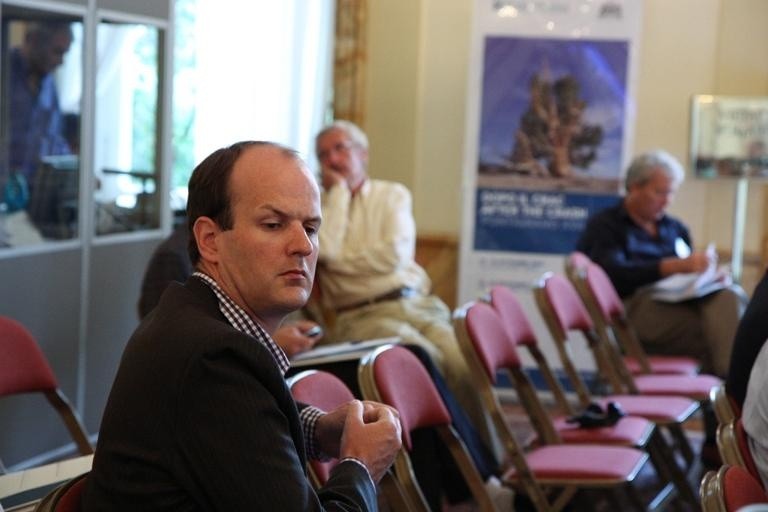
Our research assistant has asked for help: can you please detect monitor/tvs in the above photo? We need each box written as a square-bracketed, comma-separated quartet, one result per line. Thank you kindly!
[29, 154, 80, 240]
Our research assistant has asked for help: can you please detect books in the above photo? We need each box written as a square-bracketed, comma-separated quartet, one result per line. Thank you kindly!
[290, 337, 400, 367]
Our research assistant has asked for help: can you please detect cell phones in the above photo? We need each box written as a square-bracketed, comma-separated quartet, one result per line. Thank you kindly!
[304, 327, 320, 337]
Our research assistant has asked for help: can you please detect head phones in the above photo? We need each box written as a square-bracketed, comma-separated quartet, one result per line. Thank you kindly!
[566, 401, 623, 427]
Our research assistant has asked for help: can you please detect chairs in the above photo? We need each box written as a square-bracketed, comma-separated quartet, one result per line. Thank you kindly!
[484, 282, 675, 466]
[564, 253, 735, 425]
[529, 267, 706, 470]
[450, 298, 679, 512]
[282, 364, 387, 498]
[352, 339, 503, 511]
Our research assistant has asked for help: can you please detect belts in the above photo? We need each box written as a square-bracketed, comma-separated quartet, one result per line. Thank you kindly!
[335, 286, 414, 313]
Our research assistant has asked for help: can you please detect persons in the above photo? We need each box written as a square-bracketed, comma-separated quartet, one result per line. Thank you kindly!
[571, 148, 744, 381]
[725, 271, 767, 489]
[78, 140, 401, 512]
[2, 14, 75, 251]
[283, 119, 493, 454]
[136, 223, 322, 359]
